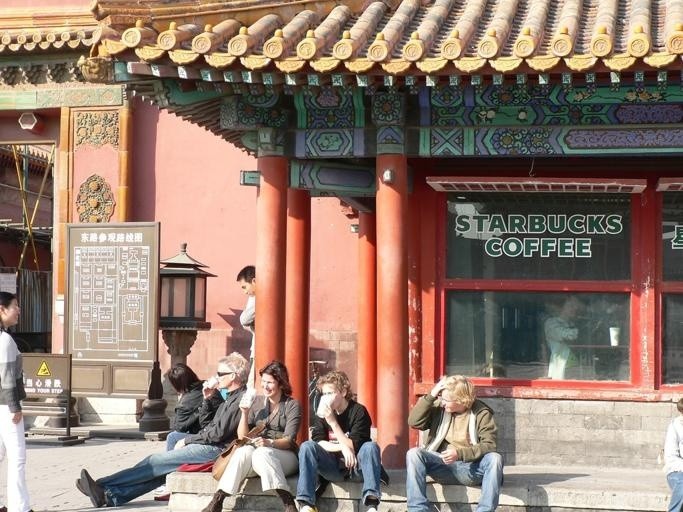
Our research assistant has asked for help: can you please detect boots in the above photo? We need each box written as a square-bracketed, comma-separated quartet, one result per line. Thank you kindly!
[202, 490, 227, 512]
[277, 489, 297, 512]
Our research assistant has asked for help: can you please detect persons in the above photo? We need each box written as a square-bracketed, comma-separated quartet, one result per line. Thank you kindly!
[237, 265, 256, 387]
[664, 398, 683, 512]
[76, 353, 251, 512]
[406, 376, 503, 512]
[202, 361, 302, 512]
[543, 299, 578, 378]
[0, 292, 34, 512]
[296, 372, 390, 512]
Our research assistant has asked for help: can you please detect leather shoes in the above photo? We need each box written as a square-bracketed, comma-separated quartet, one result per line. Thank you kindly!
[75, 469, 106, 508]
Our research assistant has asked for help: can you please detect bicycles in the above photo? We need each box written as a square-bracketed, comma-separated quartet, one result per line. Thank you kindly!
[309, 360, 328, 416]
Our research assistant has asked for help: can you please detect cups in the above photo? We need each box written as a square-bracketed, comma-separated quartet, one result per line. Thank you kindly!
[316, 395, 334, 418]
[207, 376, 219, 390]
[239, 387, 257, 408]
[610, 327, 620, 347]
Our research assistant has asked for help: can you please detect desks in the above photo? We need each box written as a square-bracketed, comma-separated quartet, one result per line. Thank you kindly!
[570, 342, 629, 380]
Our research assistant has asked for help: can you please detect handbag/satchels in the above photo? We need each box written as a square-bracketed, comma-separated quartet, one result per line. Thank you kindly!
[211, 439, 243, 481]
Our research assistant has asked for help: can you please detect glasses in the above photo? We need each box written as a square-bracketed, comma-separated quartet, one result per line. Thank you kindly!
[441, 397, 457, 403]
[218, 372, 232, 376]
